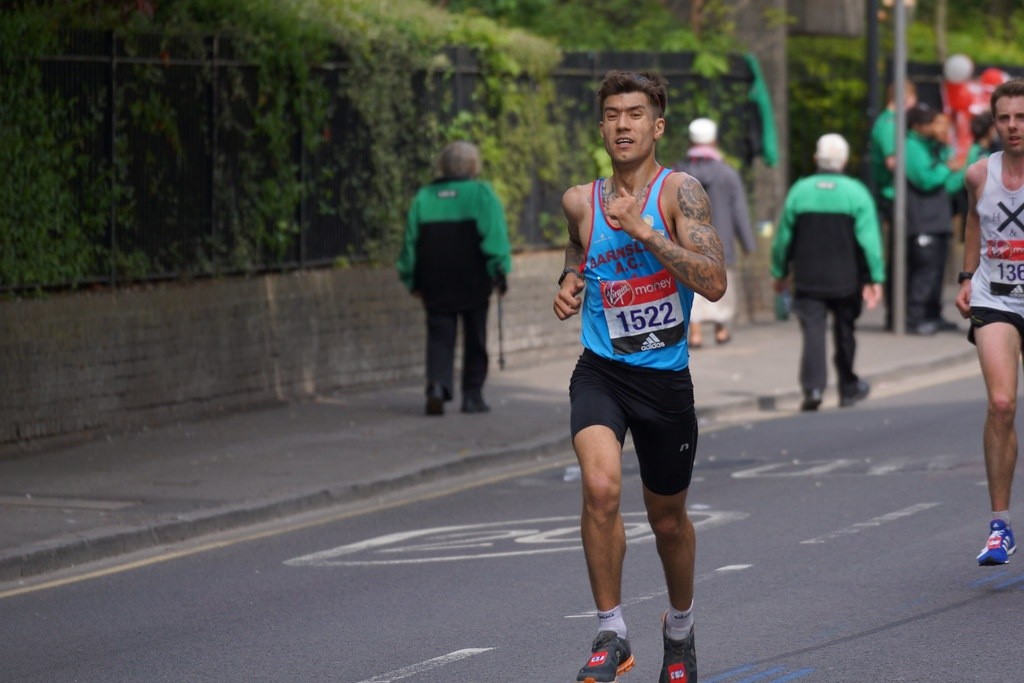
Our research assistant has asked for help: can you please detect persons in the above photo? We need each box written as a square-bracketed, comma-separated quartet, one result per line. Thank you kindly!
[771, 131, 886, 412]
[904, 104, 1003, 335]
[956, 79, 1024, 565]
[674, 118, 754, 348]
[554, 71, 728, 683]
[397, 141, 511, 415]
[870, 76, 916, 331]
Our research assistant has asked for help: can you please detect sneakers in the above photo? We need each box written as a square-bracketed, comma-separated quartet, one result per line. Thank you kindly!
[659, 609, 697, 683]
[576, 631, 636, 683]
[976, 519, 1017, 565]
[802, 389, 821, 411]
[841, 384, 869, 406]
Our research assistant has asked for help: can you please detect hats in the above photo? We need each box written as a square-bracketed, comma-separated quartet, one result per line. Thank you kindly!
[689, 119, 717, 145]
[815, 133, 849, 170]
[908, 104, 935, 127]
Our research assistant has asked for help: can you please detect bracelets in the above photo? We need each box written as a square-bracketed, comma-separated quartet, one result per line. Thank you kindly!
[558, 268, 584, 285]
[958, 271, 972, 284]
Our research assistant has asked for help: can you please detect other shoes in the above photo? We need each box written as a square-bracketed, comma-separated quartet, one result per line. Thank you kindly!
[908, 319, 958, 336]
[462, 399, 490, 413]
[427, 389, 444, 414]
